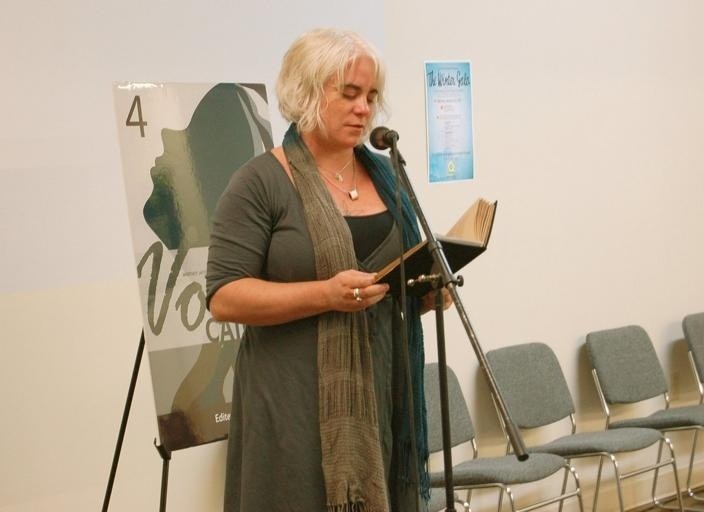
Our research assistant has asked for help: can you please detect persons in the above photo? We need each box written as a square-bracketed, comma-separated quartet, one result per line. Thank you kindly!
[204, 25, 453, 512]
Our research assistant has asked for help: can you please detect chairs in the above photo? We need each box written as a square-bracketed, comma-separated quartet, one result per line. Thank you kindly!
[485, 342, 683, 512]
[587, 326, 704, 508]
[418, 363, 583, 512]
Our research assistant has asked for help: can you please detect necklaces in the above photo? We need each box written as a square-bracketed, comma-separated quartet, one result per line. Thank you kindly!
[315, 159, 352, 182]
[317, 161, 359, 200]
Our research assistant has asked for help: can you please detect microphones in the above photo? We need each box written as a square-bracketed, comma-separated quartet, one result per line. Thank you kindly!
[370, 126, 400, 150]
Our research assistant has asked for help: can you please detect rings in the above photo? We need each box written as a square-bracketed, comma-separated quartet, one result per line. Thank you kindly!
[354, 287, 362, 302]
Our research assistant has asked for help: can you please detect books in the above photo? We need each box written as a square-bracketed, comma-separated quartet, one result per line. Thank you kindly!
[372, 199, 500, 298]
